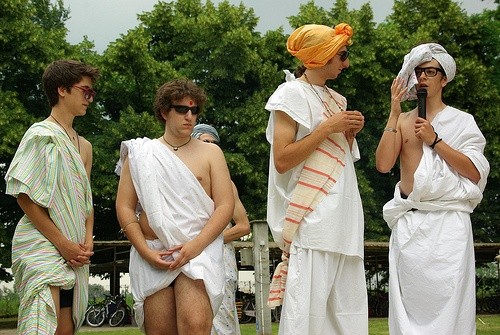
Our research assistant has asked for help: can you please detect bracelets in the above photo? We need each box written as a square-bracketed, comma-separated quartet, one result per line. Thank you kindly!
[383, 127, 397, 133]
[429, 133, 444, 149]
[124, 222, 139, 230]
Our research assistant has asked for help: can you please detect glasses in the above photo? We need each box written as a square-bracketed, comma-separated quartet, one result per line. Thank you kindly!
[414, 67, 445, 78]
[334, 51, 349, 62]
[72, 85, 96, 99]
[202, 139, 218, 145]
[170, 104, 198, 116]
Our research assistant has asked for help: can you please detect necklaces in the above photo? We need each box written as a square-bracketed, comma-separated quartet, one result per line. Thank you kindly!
[164, 136, 191, 151]
[305, 73, 356, 137]
[50, 114, 80, 153]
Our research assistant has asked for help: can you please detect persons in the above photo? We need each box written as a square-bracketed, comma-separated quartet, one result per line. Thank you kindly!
[4, 60, 101, 335]
[266, 23, 368, 335]
[376, 43, 490, 335]
[115, 78, 234, 335]
[192, 125, 250, 335]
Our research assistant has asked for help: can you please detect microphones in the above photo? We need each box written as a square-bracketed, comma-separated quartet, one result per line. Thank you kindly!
[416, 89, 427, 120]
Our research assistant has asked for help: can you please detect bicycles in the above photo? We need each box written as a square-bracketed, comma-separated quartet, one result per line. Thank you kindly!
[82, 293, 128, 328]
[239, 283, 283, 324]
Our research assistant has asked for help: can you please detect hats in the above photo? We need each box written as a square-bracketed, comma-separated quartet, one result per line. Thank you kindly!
[286, 22, 353, 68]
